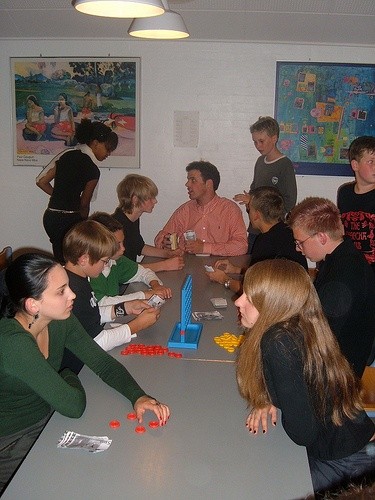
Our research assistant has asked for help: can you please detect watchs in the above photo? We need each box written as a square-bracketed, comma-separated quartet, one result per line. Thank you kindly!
[224, 276, 232, 289]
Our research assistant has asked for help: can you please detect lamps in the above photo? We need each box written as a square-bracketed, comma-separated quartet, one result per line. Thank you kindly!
[128, 0, 190, 39]
[74, 0, 165, 18]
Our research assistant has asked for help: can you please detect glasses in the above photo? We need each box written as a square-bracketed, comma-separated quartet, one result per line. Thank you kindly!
[100, 258, 111, 265]
[296, 233, 318, 250]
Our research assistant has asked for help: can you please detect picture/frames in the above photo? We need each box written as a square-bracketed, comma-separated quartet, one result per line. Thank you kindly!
[9, 56, 140, 169]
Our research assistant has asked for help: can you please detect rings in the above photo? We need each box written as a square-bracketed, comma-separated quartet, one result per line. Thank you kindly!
[156, 402, 160, 405]
[188, 251, 192, 255]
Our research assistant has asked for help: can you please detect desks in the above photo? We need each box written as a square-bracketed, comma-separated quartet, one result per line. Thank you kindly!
[0, 254, 314, 500]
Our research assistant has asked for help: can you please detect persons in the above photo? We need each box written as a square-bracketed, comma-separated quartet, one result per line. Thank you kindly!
[108, 173, 185, 275]
[35, 121, 118, 265]
[207, 188, 310, 293]
[336, 135, 375, 268]
[233, 258, 375, 500]
[285, 196, 375, 388]
[153, 160, 249, 257]
[58, 211, 173, 376]
[232, 116, 297, 255]
[0, 253, 170, 495]
